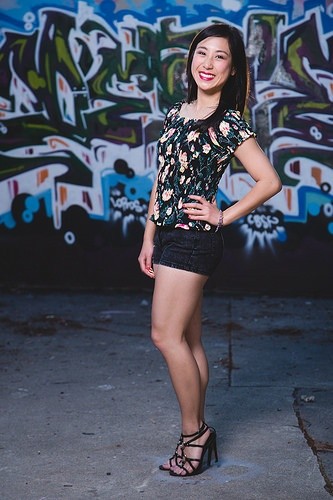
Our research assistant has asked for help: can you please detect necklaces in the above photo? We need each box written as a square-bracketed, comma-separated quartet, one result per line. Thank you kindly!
[194, 110, 215, 122]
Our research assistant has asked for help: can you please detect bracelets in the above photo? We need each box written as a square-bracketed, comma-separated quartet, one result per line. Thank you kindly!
[215, 210, 223, 232]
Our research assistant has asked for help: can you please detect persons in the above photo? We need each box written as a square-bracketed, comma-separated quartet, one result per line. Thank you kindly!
[138, 24, 282, 477]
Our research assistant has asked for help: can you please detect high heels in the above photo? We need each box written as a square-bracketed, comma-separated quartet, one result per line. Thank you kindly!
[169, 427, 218, 478]
[159, 422, 212, 471]
